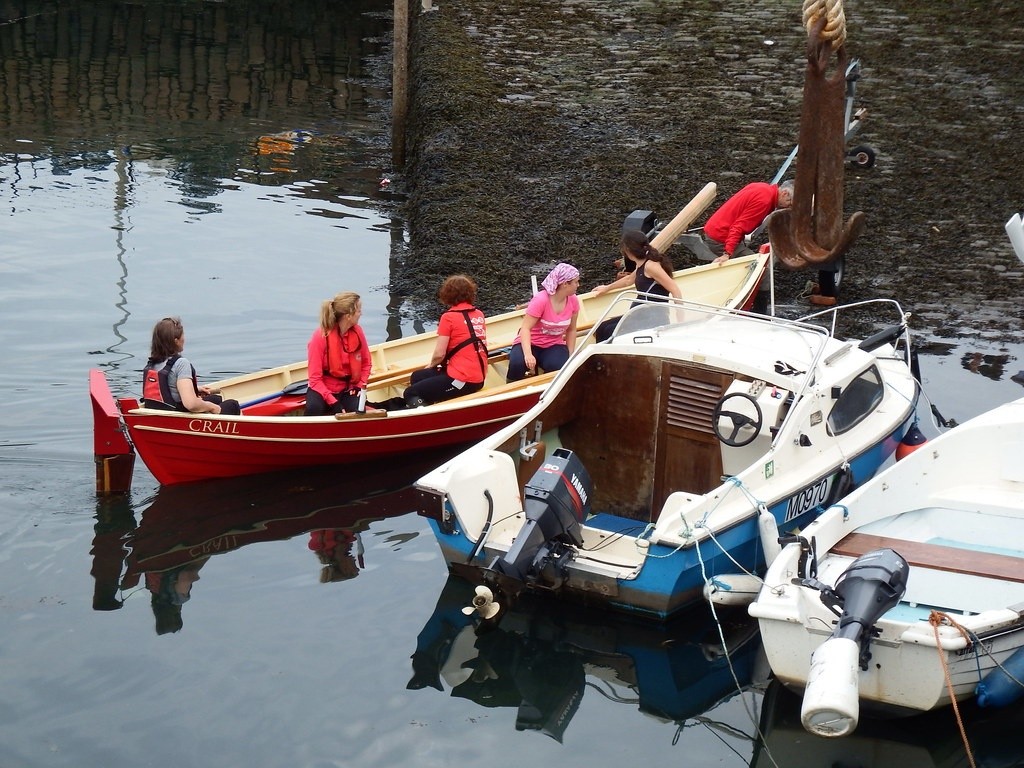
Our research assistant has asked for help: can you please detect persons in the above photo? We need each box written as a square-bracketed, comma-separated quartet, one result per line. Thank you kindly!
[595, 230, 685, 344]
[307, 528, 360, 583]
[145, 555, 208, 636]
[703, 179, 795, 265]
[303, 292, 372, 416]
[403, 275, 489, 408]
[139, 314, 244, 417]
[506, 262, 581, 384]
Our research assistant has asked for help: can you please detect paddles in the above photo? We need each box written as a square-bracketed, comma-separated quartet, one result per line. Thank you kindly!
[239, 378, 309, 409]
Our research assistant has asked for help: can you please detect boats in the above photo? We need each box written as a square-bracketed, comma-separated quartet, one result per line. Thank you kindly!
[411, 285, 923, 633]
[88, 442, 483, 639]
[401, 568, 782, 746]
[88, 251, 773, 501]
[748, 394, 1024, 738]
